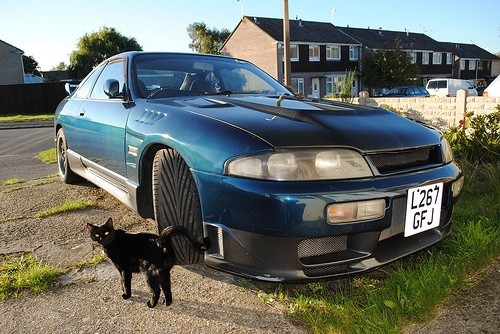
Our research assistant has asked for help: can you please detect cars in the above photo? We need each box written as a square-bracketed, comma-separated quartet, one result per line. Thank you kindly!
[376, 85, 430, 97]
[54, 50, 464, 283]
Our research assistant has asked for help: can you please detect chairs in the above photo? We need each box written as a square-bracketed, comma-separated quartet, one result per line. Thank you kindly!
[180, 73, 209, 94]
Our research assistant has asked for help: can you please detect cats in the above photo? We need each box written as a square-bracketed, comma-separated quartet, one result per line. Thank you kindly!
[87, 217, 202, 309]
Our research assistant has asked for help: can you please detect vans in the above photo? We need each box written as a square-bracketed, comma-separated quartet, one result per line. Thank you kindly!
[426, 77, 479, 97]
